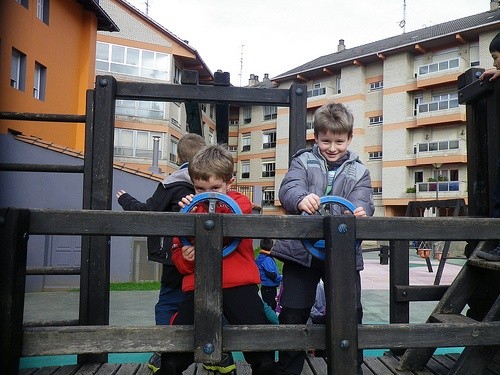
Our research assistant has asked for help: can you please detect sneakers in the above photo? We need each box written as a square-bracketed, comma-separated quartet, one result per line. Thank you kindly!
[201, 350, 237, 374]
[146, 352, 163, 375]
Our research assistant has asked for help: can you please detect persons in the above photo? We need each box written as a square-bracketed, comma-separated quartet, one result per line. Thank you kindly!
[479, 32, 500, 80]
[116, 133, 328, 375]
[274, 102, 375, 375]
